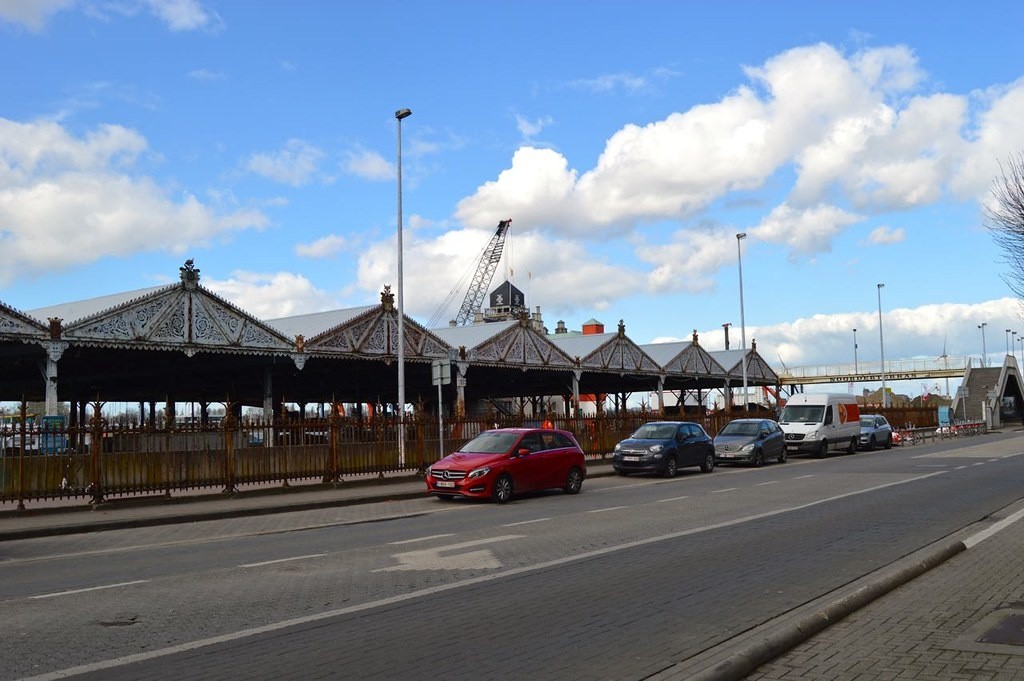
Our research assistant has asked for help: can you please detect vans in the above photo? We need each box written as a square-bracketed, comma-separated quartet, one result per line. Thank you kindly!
[777, 392, 861, 459]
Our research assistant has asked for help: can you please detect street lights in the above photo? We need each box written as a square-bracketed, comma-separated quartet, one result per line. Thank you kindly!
[1017, 337, 1024, 369]
[977, 323, 988, 367]
[877, 283, 887, 409]
[1005, 329, 1018, 356]
[395, 108, 413, 470]
[736, 232, 749, 412]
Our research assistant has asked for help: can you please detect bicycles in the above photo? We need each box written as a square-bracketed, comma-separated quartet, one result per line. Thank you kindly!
[894, 421, 922, 445]
[935, 418, 987, 438]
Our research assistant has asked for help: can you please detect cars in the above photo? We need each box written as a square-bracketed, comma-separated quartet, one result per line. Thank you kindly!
[858, 414, 893, 451]
[713, 418, 788, 468]
[424, 427, 587, 503]
[612, 421, 716, 478]
